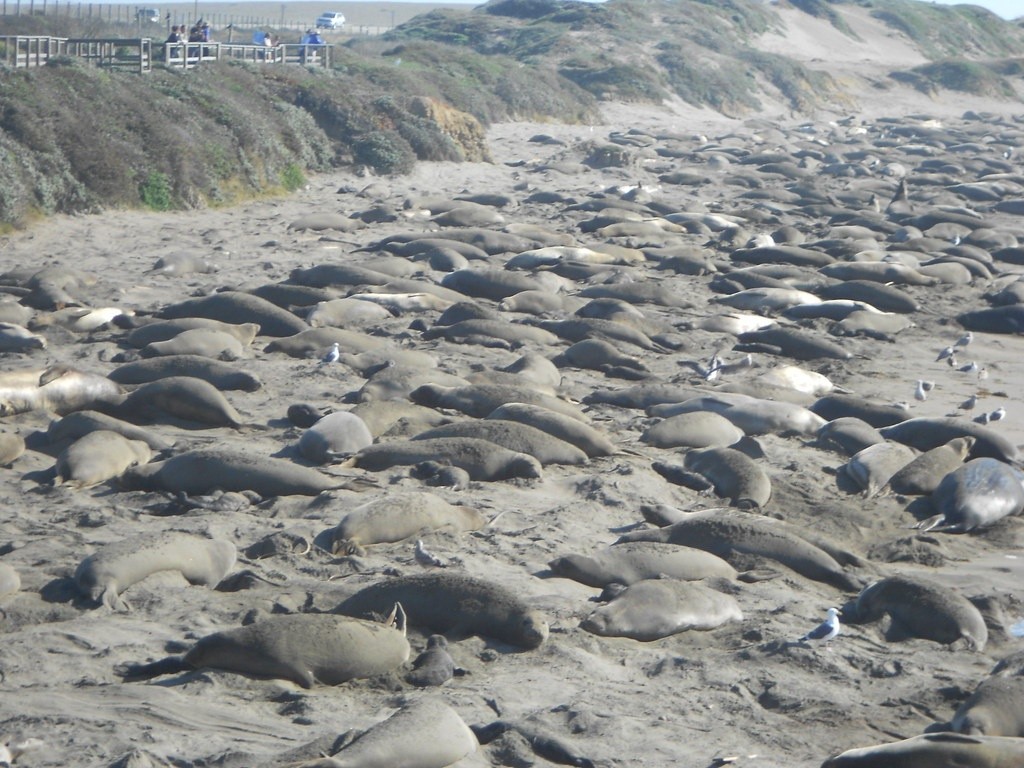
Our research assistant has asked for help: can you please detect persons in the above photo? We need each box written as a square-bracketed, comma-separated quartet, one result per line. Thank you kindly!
[188, 20, 209, 64]
[299, 29, 326, 63]
[259, 32, 280, 59]
[161, 25, 186, 66]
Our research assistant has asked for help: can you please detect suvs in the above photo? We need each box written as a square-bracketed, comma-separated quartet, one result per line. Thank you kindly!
[316, 12, 346, 30]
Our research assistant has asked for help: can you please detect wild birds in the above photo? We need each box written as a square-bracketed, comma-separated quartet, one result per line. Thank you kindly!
[934, 345, 953, 362]
[914, 380, 934, 403]
[973, 406, 1006, 425]
[413, 539, 449, 576]
[317, 342, 340, 368]
[955, 361, 978, 376]
[976, 367, 988, 381]
[952, 331, 973, 347]
[798, 608, 843, 653]
[947, 353, 956, 367]
[957, 394, 980, 412]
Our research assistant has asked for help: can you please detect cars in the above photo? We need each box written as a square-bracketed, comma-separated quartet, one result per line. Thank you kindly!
[133, 8, 160, 23]
